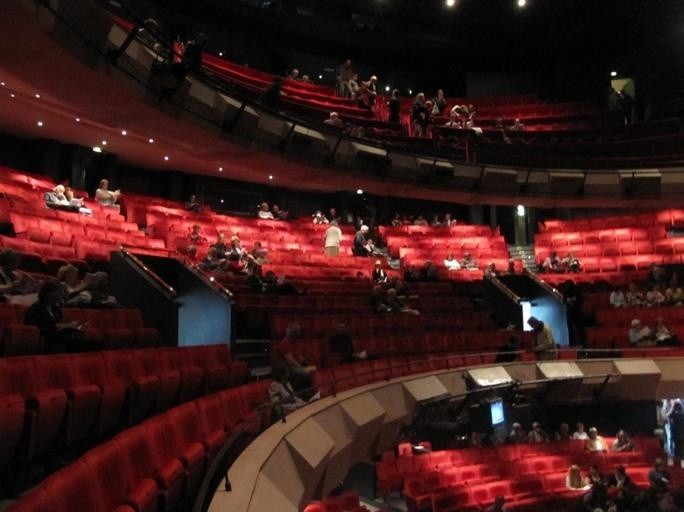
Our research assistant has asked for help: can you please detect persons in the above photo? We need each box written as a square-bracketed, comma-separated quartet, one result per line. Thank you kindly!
[369, 259, 439, 314]
[185, 224, 278, 294]
[445, 252, 516, 281]
[186, 194, 201, 213]
[1, 253, 108, 352]
[272, 319, 359, 402]
[541, 251, 582, 275]
[50, 178, 118, 215]
[610, 266, 684, 347]
[324, 220, 369, 257]
[509, 422, 684, 512]
[139, 19, 208, 69]
[290, 59, 524, 140]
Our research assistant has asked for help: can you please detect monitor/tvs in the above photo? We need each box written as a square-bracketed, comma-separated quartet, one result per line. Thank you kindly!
[468, 396, 506, 428]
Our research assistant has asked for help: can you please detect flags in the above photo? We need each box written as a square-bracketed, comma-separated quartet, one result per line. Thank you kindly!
[258, 203, 457, 226]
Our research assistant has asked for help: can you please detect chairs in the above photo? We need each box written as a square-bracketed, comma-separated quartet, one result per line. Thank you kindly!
[0, 0, 684, 512]
